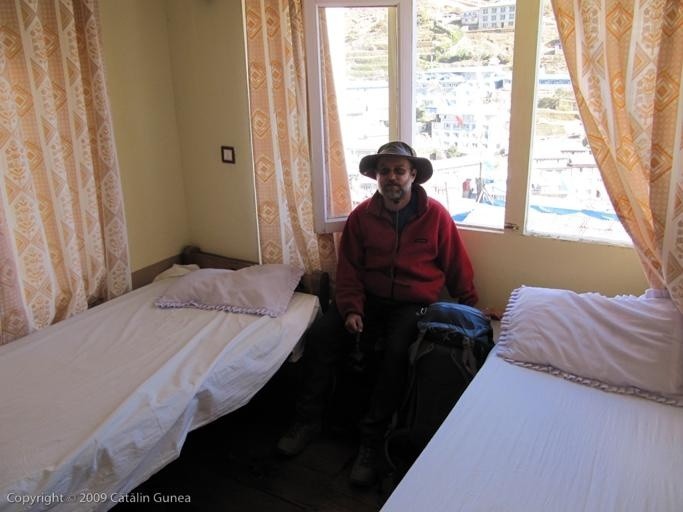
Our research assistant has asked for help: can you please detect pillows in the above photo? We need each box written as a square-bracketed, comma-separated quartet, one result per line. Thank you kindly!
[157, 265, 305, 318]
[500, 289, 679, 403]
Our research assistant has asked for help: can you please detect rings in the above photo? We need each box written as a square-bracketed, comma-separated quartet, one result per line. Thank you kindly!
[486, 307, 488, 310]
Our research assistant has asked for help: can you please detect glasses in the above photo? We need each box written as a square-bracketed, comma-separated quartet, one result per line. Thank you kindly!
[374, 165, 414, 176]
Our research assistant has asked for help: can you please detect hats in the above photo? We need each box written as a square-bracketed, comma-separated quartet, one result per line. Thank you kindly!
[359, 141, 433, 185]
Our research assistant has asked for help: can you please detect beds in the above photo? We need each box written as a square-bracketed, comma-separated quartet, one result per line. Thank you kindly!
[1, 246, 328, 511]
[380, 284, 681, 511]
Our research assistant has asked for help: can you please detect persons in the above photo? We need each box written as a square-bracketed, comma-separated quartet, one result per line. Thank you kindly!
[337, 141, 502, 495]
[462, 178, 475, 198]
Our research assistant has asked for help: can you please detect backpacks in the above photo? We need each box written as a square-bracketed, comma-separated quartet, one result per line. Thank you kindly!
[382, 301, 495, 478]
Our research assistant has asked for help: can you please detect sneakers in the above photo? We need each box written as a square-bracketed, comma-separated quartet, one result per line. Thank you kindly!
[276, 417, 324, 457]
[349, 430, 382, 486]
